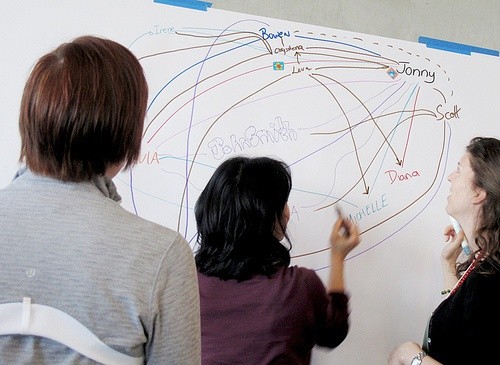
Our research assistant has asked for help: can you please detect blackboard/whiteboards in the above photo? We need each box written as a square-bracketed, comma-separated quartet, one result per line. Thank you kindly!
[0, 1, 500, 365]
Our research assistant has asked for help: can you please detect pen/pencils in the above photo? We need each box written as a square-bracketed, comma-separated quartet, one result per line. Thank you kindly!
[334, 204, 349, 236]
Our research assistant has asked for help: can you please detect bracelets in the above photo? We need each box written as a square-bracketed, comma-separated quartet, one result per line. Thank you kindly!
[440, 289, 451, 295]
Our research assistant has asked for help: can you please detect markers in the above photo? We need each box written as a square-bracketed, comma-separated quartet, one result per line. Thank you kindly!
[449, 215, 471, 256]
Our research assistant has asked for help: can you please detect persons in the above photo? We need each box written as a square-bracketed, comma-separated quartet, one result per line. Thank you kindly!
[1, 35, 202, 365]
[193, 157, 361, 365]
[384, 137, 500, 365]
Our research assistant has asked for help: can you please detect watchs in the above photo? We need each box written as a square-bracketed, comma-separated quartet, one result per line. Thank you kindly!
[409, 351, 428, 365]
